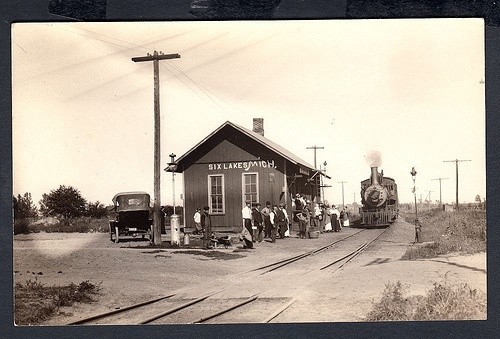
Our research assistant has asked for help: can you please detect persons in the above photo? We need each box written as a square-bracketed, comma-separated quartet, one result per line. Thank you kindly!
[291, 192, 350, 239]
[242, 200, 255, 243]
[253, 203, 264, 243]
[268, 205, 278, 243]
[201, 206, 214, 250]
[161, 206, 167, 234]
[261, 201, 272, 239]
[277, 203, 292, 239]
[194, 207, 201, 234]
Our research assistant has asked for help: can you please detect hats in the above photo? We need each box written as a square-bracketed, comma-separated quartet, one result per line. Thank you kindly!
[203, 205, 209, 210]
[266, 201, 270, 205]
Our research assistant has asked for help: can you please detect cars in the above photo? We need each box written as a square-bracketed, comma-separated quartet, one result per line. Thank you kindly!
[110, 192, 154, 243]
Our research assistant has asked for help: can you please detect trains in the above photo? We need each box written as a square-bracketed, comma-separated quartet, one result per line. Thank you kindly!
[356, 166, 399, 228]
